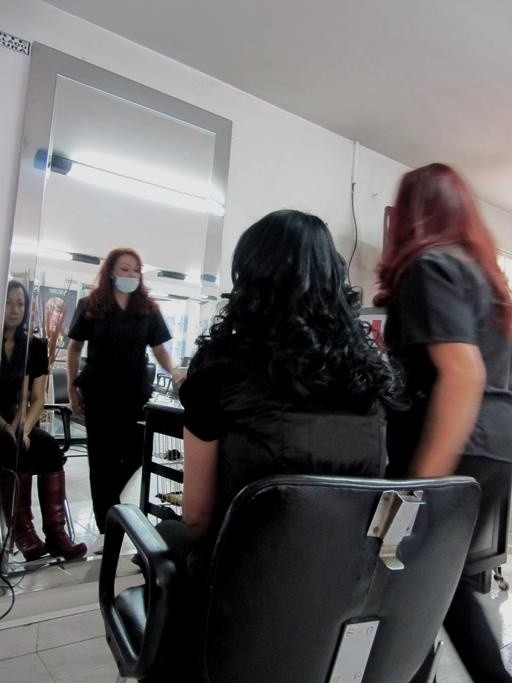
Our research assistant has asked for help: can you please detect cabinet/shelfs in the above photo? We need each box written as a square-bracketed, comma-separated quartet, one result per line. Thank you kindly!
[140, 402, 184, 519]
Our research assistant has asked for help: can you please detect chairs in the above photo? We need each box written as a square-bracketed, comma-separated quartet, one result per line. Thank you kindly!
[1, 400, 75, 566]
[96, 475, 483, 682]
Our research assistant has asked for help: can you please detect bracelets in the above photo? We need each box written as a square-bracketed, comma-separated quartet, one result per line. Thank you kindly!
[4, 424, 11, 432]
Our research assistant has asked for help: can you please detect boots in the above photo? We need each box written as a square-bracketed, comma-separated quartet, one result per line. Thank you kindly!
[0, 470, 88, 562]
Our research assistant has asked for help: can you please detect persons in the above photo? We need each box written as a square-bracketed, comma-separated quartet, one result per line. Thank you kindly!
[67, 249, 187, 556]
[32, 296, 69, 349]
[178, 209, 412, 566]
[0, 281, 87, 561]
[374, 164, 512, 683]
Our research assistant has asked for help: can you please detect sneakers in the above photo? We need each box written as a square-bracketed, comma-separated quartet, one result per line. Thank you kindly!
[91, 535, 105, 554]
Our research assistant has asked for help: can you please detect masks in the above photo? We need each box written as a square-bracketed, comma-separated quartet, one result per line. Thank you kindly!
[111, 272, 140, 294]
